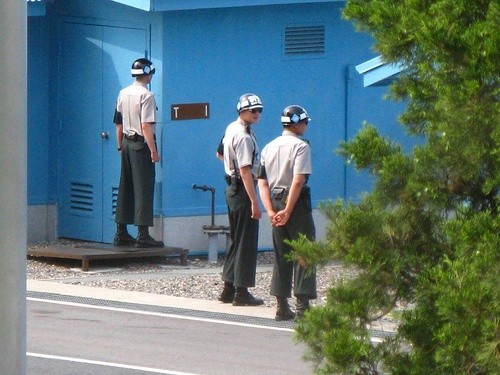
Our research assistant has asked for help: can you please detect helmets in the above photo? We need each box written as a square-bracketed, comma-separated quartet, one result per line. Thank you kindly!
[237, 93, 264, 112]
[131, 58, 155, 77]
[281, 105, 311, 125]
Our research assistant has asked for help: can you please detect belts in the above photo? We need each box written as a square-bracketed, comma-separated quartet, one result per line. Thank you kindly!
[123, 133, 156, 141]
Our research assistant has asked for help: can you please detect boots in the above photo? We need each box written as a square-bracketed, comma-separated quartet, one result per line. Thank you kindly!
[294, 295, 312, 321]
[113, 223, 137, 246]
[275, 295, 295, 321]
[221, 282, 236, 303]
[137, 226, 164, 248]
[232, 287, 264, 306]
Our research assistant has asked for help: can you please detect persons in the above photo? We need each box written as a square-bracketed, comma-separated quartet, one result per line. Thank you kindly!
[113, 57, 166, 249]
[257, 105, 319, 322]
[216, 93, 265, 307]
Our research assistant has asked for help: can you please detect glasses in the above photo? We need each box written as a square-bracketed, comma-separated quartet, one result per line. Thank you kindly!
[302, 120, 308, 125]
[244, 108, 263, 113]
